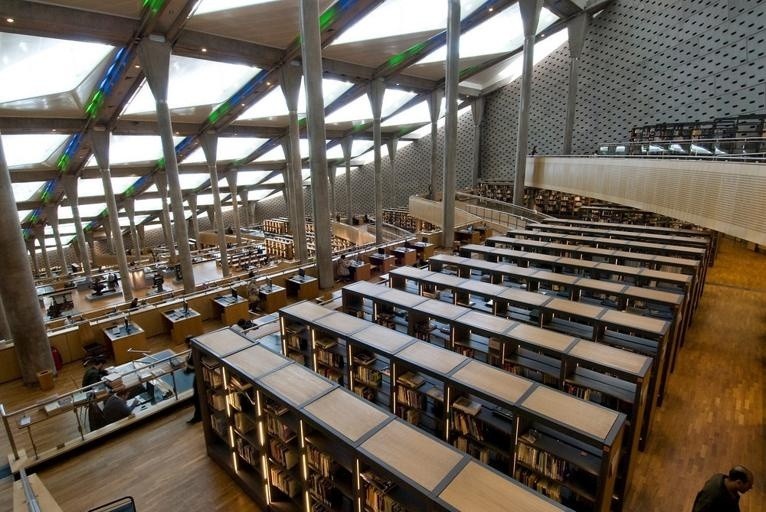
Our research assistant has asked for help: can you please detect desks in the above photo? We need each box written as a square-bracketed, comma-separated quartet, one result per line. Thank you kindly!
[100, 306, 204, 415]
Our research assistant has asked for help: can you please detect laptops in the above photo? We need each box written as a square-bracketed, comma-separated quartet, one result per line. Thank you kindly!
[134, 381, 154, 404]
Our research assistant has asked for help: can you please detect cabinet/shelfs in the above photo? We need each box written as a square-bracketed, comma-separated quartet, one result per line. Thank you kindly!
[631, 113, 766, 163]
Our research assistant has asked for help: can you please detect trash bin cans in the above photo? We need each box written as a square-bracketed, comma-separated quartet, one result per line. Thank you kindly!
[36, 368, 54, 391]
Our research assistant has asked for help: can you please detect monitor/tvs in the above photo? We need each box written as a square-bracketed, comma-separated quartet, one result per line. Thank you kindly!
[231, 287, 238, 301]
[182, 300, 190, 315]
[404, 242, 410, 248]
[299, 269, 304, 277]
[124, 315, 131, 332]
[266, 277, 272, 288]
[378, 248, 384, 254]
[422, 237, 428, 242]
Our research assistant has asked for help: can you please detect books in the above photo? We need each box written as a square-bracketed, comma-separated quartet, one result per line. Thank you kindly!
[283, 317, 566, 504]
[347, 277, 619, 409]
[200, 351, 433, 512]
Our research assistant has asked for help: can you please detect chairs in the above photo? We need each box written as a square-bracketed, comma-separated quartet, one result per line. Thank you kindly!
[89, 402, 108, 431]
[70, 374, 90, 428]
[78, 321, 110, 367]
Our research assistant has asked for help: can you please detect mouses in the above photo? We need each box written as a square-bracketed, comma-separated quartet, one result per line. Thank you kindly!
[175, 317, 179, 320]
[232, 301, 235, 303]
[116, 336, 120, 337]
[300, 280, 303, 282]
[268, 289, 271, 291]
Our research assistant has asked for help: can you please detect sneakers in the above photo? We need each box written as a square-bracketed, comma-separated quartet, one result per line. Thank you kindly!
[187, 417, 202, 423]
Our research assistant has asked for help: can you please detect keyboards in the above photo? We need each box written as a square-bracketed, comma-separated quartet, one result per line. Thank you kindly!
[112, 325, 120, 334]
[175, 312, 184, 318]
[224, 298, 230, 303]
[262, 287, 268, 290]
[293, 277, 301, 281]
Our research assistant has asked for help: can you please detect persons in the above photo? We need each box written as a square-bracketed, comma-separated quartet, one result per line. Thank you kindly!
[227, 243, 234, 249]
[64, 316, 73, 329]
[131, 298, 138, 309]
[130, 260, 135, 266]
[81, 334, 201, 424]
[336, 214, 341, 221]
[337, 255, 352, 275]
[691, 464, 754, 511]
[108, 273, 118, 287]
